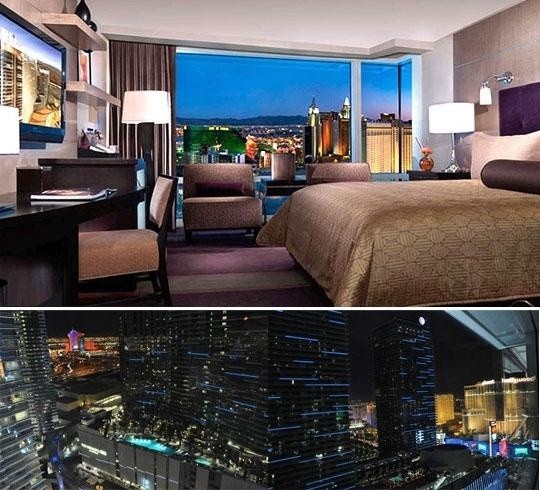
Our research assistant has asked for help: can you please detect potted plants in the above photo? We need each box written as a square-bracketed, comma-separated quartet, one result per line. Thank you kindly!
[257, 142, 295, 181]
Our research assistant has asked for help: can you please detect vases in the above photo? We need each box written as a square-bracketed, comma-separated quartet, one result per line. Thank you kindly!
[75, 0, 91, 24]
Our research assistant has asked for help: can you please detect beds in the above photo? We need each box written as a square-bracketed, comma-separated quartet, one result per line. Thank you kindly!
[255, 82, 539, 307]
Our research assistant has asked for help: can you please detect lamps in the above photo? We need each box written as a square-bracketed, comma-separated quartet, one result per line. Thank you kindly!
[428, 103, 475, 172]
[120, 91, 174, 228]
[0, 105, 20, 213]
[479, 71, 514, 106]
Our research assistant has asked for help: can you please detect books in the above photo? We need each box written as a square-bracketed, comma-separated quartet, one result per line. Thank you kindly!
[29, 186, 118, 200]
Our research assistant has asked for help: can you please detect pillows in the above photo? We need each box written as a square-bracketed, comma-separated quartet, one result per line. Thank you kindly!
[481, 159, 540, 194]
[464, 131, 540, 179]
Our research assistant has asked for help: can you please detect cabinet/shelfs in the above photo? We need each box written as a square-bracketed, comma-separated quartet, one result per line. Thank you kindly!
[38, 158, 148, 232]
[42, 12, 123, 108]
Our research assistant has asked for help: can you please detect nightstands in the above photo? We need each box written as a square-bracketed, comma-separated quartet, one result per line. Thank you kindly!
[406, 169, 471, 180]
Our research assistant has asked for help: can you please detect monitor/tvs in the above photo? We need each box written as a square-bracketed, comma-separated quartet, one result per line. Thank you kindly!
[0, 3, 67, 149]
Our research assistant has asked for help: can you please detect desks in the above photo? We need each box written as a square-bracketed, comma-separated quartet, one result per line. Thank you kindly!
[0, 186, 146, 307]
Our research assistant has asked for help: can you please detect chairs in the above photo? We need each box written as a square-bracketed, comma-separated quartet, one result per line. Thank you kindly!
[78, 174, 178, 307]
[183, 163, 264, 241]
[304, 162, 371, 186]
